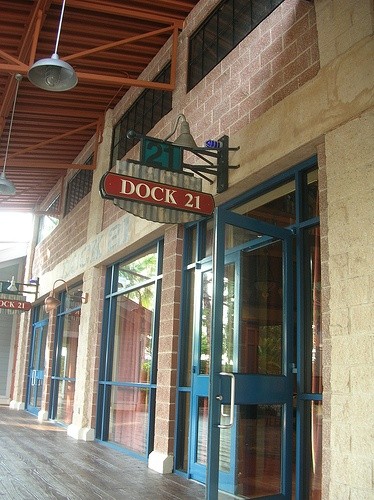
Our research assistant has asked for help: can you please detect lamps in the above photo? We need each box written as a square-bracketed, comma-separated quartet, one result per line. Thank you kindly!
[204, 139, 223, 149]
[27, 0, 78, 92]
[29, 279, 36, 283]
[164, 114, 198, 149]
[44, 279, 88, 313]
[7, 276, 17, 291]
[0, 73, 23, 196]
